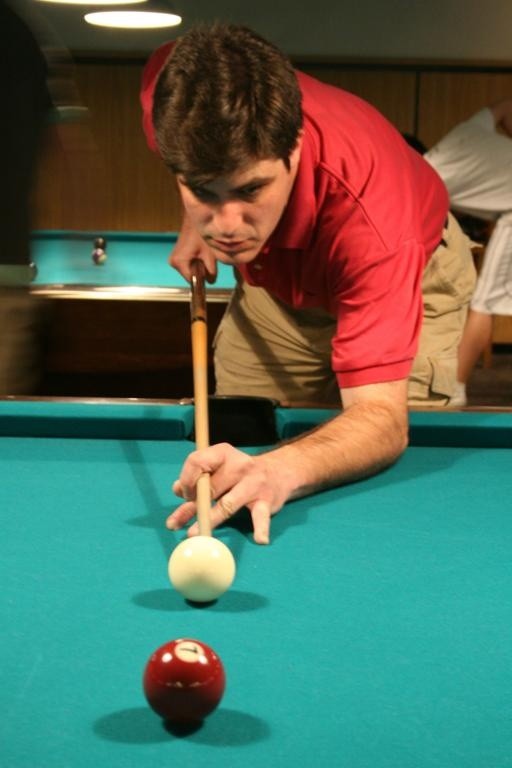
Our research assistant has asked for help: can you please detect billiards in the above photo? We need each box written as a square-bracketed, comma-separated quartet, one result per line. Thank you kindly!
[142, 638, 228, 726]
[165, 537, 238, 603]
[92, 248, 107, 264]
[93, 236, 107, 249]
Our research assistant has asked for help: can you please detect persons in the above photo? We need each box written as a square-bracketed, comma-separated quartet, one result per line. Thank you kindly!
[137, 17, 481, 546]
[397, 101, 512, 408]
[0, 0, 56, 397]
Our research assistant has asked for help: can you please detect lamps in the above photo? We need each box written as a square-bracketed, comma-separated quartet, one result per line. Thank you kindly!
[84, 1, 182, 31]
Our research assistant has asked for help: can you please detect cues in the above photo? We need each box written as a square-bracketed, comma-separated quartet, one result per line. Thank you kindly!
[190, 258, 213, 531]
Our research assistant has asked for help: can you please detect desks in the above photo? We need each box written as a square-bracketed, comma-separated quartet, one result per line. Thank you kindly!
[28, 230, 251, 374]
[0, 397, 511, 767]
